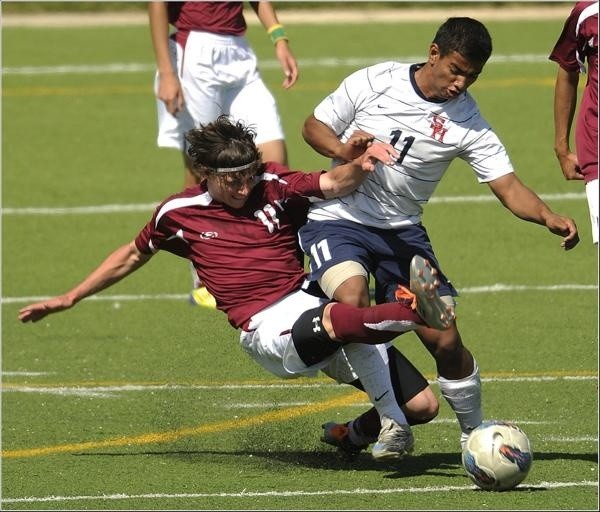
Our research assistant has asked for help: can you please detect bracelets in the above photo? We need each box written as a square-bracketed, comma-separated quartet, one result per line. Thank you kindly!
[266, 22, 290, 47]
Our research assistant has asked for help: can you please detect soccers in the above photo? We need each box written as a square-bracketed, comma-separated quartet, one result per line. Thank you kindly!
[462, 419, 532, 490]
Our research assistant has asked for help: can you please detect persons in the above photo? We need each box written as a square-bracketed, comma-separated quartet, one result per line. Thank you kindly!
[295, 15, 582, 467]
[16, 114, 457, 457]
[146, 2, 301, 311]
[545, 1, 598, 257]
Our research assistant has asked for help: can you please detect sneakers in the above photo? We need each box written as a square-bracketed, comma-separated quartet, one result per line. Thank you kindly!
[409, 254, 455, 331]
[372, 420, 414, 462]
[320, 422, 369, 451]
